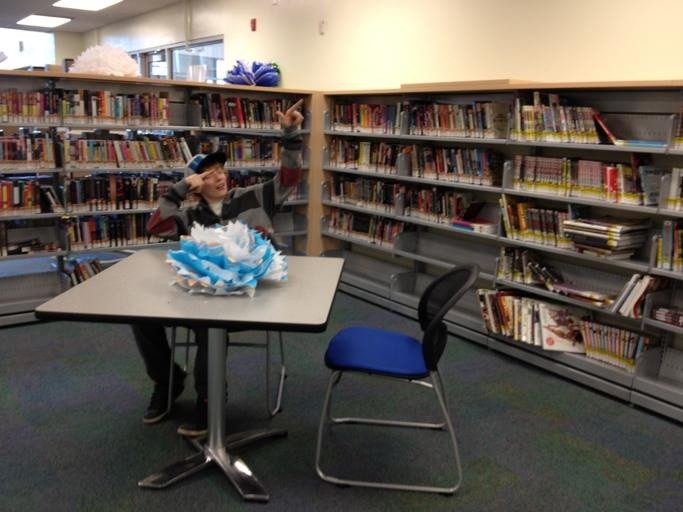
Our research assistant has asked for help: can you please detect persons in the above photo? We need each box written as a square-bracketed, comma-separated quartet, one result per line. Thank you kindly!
[131, 98, 305, 437]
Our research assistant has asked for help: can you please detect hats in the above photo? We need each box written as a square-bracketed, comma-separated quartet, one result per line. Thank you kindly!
[183, 151, 228, 180]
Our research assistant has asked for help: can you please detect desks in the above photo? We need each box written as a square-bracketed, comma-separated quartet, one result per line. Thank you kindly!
[34, 246, 347, 500]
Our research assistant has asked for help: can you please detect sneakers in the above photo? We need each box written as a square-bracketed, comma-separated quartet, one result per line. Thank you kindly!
[142, 378, 183, 423]
[177, 397, 208, 436]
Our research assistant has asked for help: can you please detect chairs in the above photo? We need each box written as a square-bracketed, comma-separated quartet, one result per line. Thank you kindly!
[314, 261, 479, 495]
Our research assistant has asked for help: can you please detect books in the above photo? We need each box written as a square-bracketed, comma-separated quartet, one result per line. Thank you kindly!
[477, 97, 682, 373]
[328, 99, 509, 247]
[0, 89, 306, 286]
